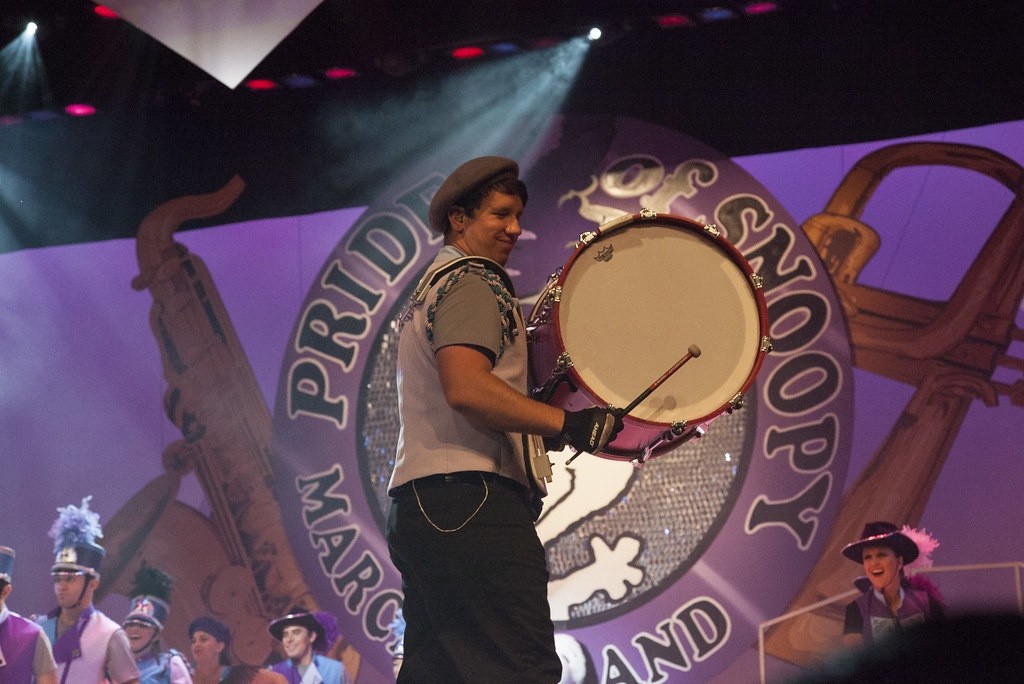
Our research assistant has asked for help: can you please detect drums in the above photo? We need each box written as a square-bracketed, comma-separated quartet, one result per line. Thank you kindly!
[528, 209, 772, 468]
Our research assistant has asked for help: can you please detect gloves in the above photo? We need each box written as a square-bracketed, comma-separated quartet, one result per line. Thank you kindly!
[563, 405, 625, 457]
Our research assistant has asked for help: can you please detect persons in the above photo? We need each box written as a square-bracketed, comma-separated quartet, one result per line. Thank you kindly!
[387, 157, 624, 684]
[841, 521, 944, 653]
[0, 545, 352, 684]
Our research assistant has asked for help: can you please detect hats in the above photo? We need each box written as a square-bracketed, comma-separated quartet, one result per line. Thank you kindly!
[428, 154, 520, 234]
[1, 545, 17, 584]
[267, 606, 340, 657]
[121, 560, 174, 633]
[188, 616, 233, 641]
[841, 521, 920, 565]
[47, 495, 106, 578]
